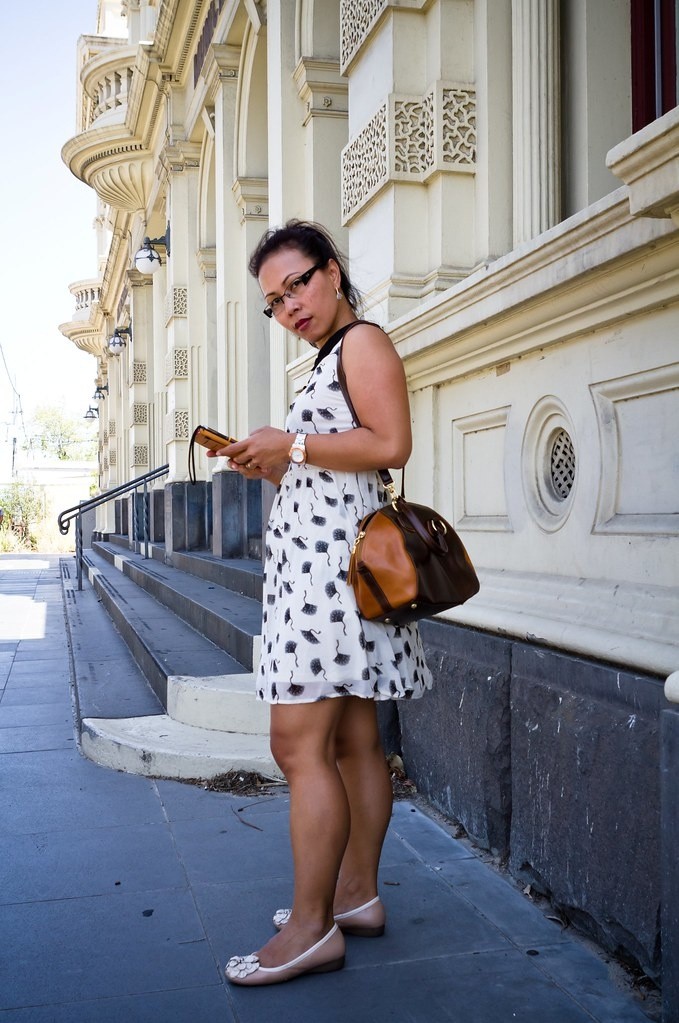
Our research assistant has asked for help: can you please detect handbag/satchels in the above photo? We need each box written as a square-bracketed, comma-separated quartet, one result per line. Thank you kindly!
[346, 500, 480, 625]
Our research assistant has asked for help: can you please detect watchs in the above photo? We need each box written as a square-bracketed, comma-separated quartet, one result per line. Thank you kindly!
[289, 432, 309, 468]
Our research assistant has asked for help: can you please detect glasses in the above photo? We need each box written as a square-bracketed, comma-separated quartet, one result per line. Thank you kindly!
[263, 263, 321, 318]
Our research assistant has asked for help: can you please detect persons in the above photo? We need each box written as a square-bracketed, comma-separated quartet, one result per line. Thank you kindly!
[223, 221, 434, 985]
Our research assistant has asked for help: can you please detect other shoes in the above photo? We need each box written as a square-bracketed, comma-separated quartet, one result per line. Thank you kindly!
[272, 895, 386, 937]
[224, 922, 346, 986]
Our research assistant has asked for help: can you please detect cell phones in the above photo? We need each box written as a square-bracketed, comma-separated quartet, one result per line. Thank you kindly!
[195, 425, 240, 452]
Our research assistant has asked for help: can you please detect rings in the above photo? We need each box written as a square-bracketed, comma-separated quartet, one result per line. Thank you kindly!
[245, 463, 252, 469]
